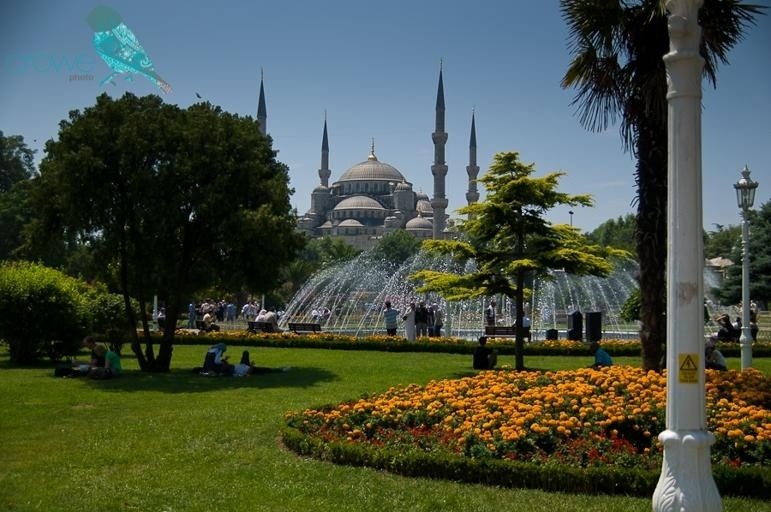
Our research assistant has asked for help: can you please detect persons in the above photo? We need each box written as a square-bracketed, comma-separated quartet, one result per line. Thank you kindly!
[83, 336, 106, 377]
[471, 336, 499, 371]
[198, 342, 231, 378]
[234, 350, 256, 376]
[482, 299, 498, 340]
[400, 299, 445, 343]
[704, 297, 760, 372]
[156, 297, 335, 332]
[511, 310, 532, 327]
[589, 340, 614, 369]
[92, 345, 123, 380]
[381, 300, 402, 337]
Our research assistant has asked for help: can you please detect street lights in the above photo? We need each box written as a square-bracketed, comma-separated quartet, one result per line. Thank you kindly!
[569, 210, 575, 225]
[733, 164, 759, 369]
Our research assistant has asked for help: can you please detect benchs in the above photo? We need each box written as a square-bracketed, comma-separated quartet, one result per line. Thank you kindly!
[195, 320, 208, 331]
[246, 321, 283, 334]
[288, 322, 321, 334]
[483, 325, 532, 342]
